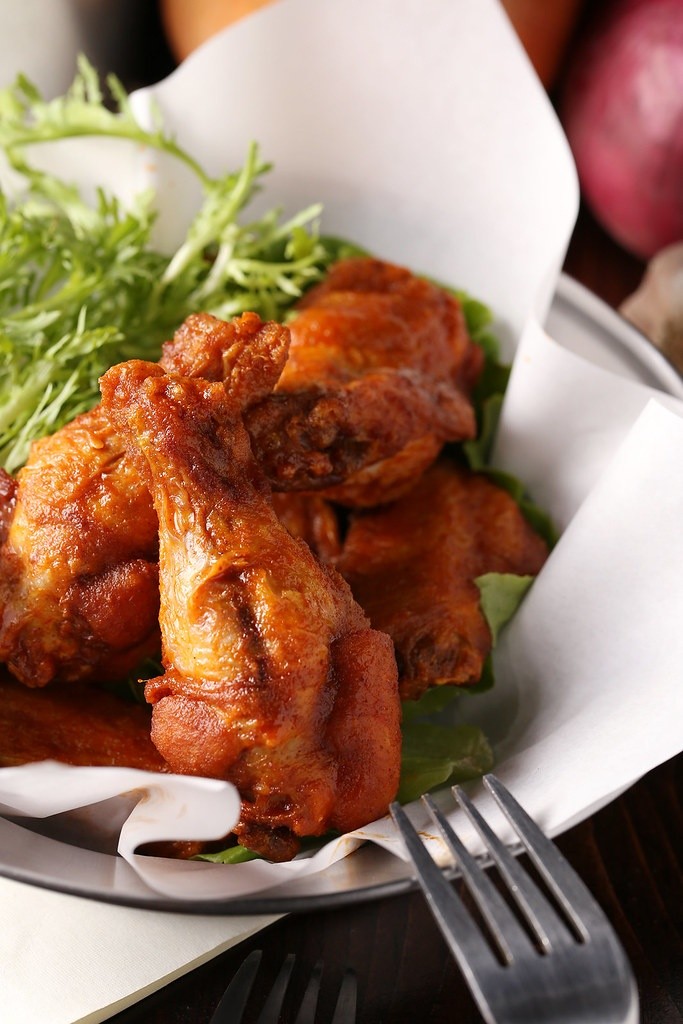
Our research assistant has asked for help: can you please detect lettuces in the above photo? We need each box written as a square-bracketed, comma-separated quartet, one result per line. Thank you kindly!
[191, 236, 550, 864]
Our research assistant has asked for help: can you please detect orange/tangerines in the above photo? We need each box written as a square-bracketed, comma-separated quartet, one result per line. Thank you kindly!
[161, 0, 580, 93]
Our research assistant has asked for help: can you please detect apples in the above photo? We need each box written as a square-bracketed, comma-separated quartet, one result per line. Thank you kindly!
[552, 0, 682, 269]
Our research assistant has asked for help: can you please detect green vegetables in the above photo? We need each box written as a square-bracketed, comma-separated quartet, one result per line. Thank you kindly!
[0, 52, 339, 473]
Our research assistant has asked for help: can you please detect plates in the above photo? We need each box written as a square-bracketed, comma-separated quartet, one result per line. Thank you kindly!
[0, 271, 683, 914]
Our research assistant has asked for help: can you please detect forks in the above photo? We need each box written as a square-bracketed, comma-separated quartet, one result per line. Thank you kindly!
[387, 774, 640, 1024]
